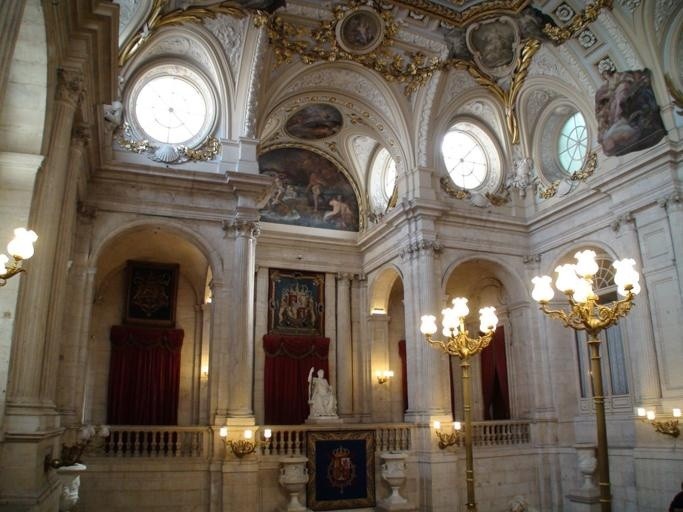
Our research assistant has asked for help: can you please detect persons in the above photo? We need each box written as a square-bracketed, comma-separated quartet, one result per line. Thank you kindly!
[325, 199, 354, 224]
[307, 368, 335, 412]
[306, 174, 324, 212]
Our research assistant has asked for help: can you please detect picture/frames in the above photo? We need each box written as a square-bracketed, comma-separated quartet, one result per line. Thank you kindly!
[304, 427, 377, 511]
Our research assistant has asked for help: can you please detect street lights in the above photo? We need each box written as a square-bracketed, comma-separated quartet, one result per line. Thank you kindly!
[530, 249, 642, 512]
[420, 296, 500, 512]
